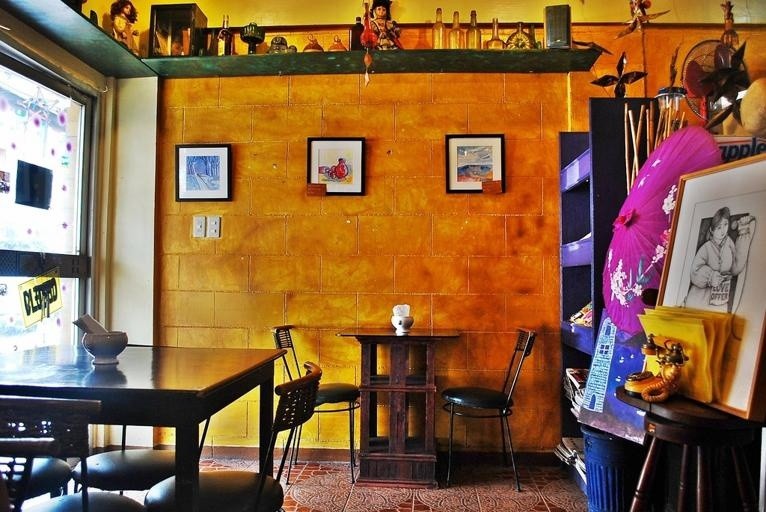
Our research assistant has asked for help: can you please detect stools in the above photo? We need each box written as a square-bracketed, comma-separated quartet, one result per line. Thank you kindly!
[50, 494, 145, 512]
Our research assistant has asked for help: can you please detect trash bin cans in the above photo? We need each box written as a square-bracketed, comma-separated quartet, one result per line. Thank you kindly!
[581, 424, 652, 512]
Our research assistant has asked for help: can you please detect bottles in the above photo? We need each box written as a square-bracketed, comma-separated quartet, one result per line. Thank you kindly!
[432, 9, 447, 50]
[506, 22, 535, 49]
[328, 35, 348, 51]
[466, 9, 482, 50]
[487, 19, 505, 50]
[303, 39, 324, 53]
[216, 14, 233, 56]
[449, 11, 464, 50]
[348, 17, 364, 50]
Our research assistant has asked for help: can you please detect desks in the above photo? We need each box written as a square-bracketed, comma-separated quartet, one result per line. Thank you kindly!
[614, 381, 760, 512]
[0, 343, 286, 511]
[335, 328, 462, 487]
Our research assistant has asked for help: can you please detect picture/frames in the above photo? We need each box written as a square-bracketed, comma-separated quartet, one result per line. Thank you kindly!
[306, 135, 366, 198]
[653, 151, 765, 423]
[174, 142, 231, 203]
[446, 132, 505, 193]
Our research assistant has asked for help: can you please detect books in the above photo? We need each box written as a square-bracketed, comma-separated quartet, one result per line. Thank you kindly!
[553, 368, 591, 485]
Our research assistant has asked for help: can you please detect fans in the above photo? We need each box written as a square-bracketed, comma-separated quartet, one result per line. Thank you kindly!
[681, 39, 751, 120]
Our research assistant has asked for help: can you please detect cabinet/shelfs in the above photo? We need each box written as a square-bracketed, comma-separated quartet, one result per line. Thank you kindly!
[559, 97, 658, 498]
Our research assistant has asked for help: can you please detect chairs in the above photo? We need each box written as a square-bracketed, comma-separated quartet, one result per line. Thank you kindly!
[0, 392, 101, 512]
[441, 327, 536, 492]
[147, 361, 322, 512]
[271, 324, 359, 486]
[71, 414, 211, 497]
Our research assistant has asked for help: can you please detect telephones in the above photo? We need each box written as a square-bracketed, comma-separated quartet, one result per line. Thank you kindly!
[626, 333, 689, 402]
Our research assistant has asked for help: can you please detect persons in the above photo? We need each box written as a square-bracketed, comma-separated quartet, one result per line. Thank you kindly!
[369, 0, 404, 51]
[110, 0, 139, 58]
[684, 207, 751, 313]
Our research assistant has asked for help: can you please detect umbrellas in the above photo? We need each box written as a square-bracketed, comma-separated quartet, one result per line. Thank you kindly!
[602, 125, 726, 336]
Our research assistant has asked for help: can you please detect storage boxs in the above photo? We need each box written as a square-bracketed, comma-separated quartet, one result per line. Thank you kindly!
[147, 2, 207, 57]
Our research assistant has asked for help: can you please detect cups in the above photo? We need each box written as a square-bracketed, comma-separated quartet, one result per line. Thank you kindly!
[82, 331, 128, 364]
[391, 316, 414, 333]
